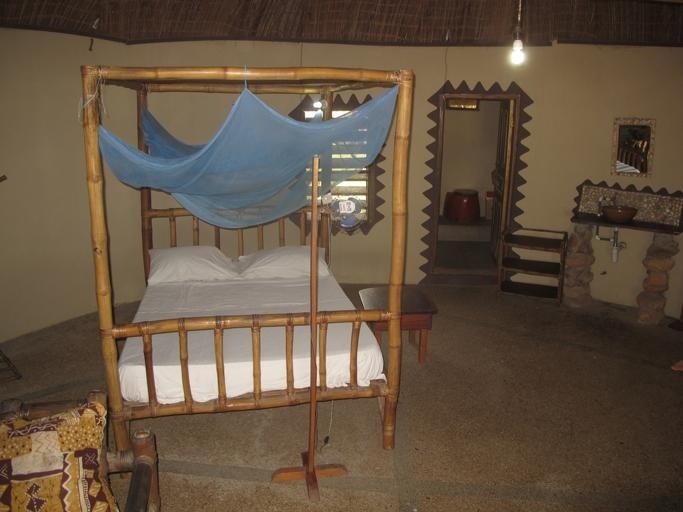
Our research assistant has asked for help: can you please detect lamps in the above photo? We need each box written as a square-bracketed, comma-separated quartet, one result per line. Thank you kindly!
[506, 0, 527, 67]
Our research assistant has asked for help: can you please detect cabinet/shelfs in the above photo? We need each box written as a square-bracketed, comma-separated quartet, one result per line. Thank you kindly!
[496, 228, 568, 305]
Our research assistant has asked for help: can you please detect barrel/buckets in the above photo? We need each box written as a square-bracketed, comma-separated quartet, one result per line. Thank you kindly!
[449, 190, 481, 218]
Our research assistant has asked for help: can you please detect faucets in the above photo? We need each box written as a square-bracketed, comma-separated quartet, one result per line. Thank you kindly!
[596, 195, 616, 242]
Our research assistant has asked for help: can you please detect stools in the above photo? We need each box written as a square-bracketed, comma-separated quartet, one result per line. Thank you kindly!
[357, 285, 439, 365]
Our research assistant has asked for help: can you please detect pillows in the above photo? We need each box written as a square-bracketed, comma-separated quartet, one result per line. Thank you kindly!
[236, 244, 331, 280]
[145, 243, 230, 285]
[0, 389, 121, 512]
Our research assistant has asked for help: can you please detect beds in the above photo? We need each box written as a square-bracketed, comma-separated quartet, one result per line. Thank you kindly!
[77, 61, 416, 452]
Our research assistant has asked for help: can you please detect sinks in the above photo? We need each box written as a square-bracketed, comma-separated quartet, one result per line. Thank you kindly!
[603, 205, 637, 224]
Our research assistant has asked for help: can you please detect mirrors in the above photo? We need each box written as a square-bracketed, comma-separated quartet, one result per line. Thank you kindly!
[609, 117, 657, 178]
